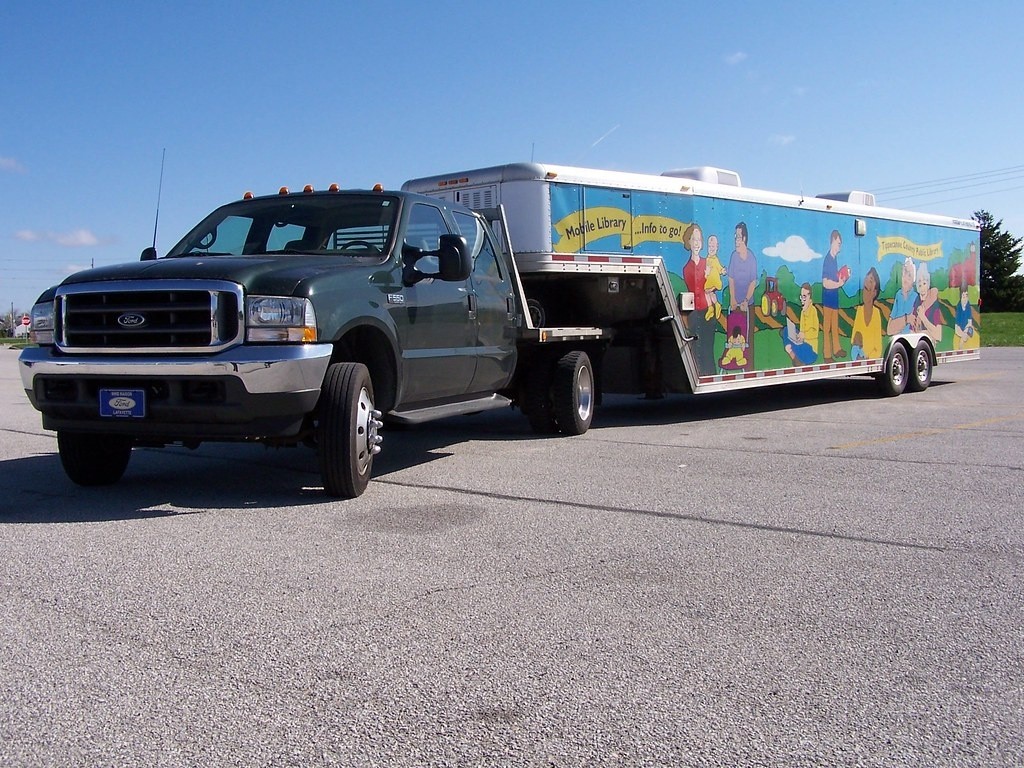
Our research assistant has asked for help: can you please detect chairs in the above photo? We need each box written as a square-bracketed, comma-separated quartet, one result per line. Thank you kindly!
[284, 240, 316, 252]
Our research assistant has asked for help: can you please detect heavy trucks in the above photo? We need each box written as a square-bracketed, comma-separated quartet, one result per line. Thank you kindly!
[18, 162, 983, 499]
